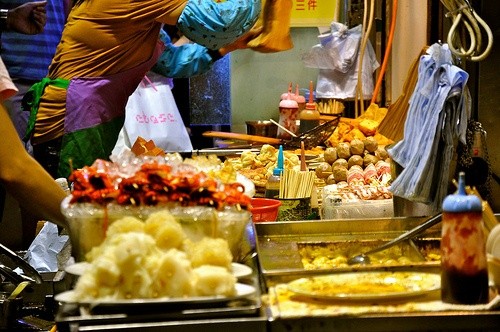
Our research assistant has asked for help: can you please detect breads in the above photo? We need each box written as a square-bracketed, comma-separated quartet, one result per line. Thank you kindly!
[337, 118, 382, 142]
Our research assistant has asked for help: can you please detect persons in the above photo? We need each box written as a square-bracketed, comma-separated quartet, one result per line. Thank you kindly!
[0, 58, 68, 230]
[1, 0, 73, 158]
[0, 0, 48, 35]
[22, 0, 265, 252]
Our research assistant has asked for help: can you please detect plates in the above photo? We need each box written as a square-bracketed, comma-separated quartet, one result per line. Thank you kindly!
[288, 271, 442, 302]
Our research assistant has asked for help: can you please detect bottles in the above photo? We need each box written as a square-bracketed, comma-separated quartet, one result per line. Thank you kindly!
[276, 83, 306, 137]
[439, 171, 488, 305]
[264, 145, 287, 199]
[300, 81, 320, 134]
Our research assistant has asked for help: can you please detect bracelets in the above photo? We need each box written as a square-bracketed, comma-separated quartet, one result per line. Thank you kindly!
[1, 9, 9, 33]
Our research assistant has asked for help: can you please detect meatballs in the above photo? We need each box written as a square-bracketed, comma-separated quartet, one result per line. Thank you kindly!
[315, 137, 389, 185]
